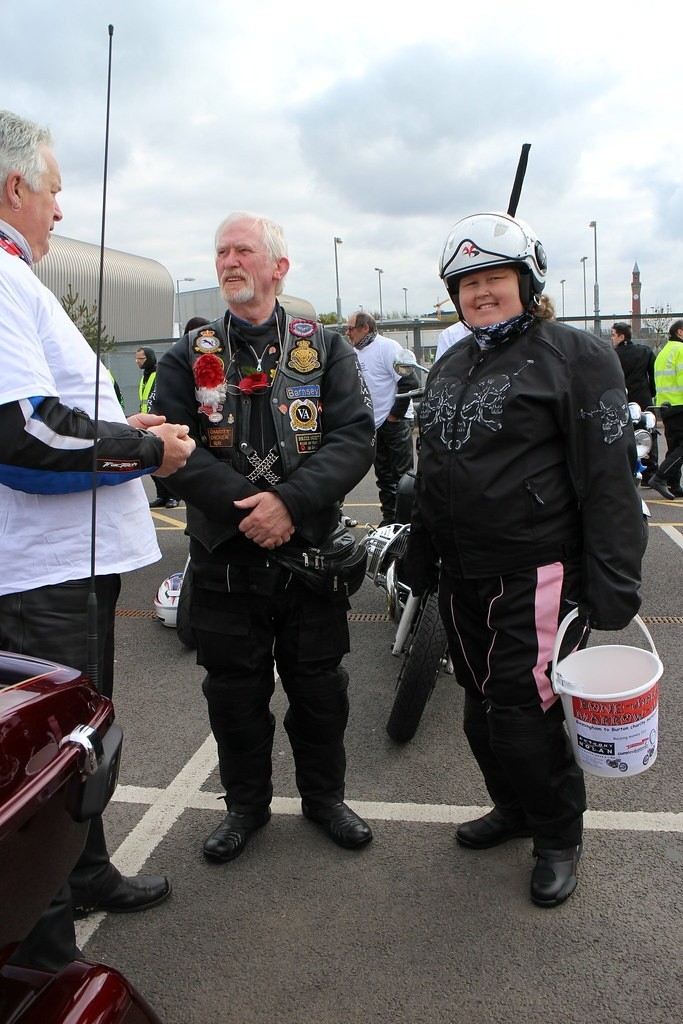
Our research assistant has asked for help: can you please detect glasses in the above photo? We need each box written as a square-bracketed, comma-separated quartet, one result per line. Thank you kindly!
[346, 326, 362, 332]
[136, 357, 147, 362]
[223, 351, 282, 396]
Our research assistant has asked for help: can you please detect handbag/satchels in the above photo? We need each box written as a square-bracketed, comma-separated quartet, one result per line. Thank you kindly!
[264, 518, 369, 603]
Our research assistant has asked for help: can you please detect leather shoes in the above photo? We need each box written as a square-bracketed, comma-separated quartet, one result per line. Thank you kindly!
[648, 475, 676, 501]
[668, 483, 683, 497]
[455, 808, 536, 849]
[165, 499, 179, 508]
[530, 842, 583, 907]
[205, 806, 271, 860]
[302, 800, 373, 848]
[150, 497, 165, 508]
[72, 874, 172, 921]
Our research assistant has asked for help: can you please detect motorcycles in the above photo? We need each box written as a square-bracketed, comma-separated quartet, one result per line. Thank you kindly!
[361, 347, 453, 745]
[0, 648, 163, 1024]
[627, 402, 671, 558]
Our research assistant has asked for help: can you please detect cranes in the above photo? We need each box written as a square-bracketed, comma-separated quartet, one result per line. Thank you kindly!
[433, 297, 450, 319]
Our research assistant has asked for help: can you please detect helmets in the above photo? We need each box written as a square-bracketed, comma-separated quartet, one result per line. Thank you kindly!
[154, 573, 184, 628]
[438, 211, 547, 322]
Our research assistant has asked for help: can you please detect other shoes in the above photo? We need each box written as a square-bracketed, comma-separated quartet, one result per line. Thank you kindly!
[641, 478, 650, 486]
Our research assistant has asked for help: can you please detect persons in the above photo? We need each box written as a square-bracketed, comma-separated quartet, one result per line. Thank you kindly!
[0, 112, 195, 919]
[395, 212, 646, 909]
[108, 316, 211, 508]
[347, 312, 420, 527]
[611, 323, 658, 485]
[648, 319, 683, 499]
[157, 213, 376, 865]
[436, 321, 475, 362]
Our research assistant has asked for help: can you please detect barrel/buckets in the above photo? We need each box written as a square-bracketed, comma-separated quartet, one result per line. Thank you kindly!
[551, 606, 664, 777]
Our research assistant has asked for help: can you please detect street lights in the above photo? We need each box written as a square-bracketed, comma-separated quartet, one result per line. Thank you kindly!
[580, 256, 589, 331]
[177, 278, 195, 338]
[560, 279, 565, 323]
[375, 267, 384, 325]
[589, 220, 599, 337]
[403, 287, 409, 348]
[334, 237, 343, 334]
